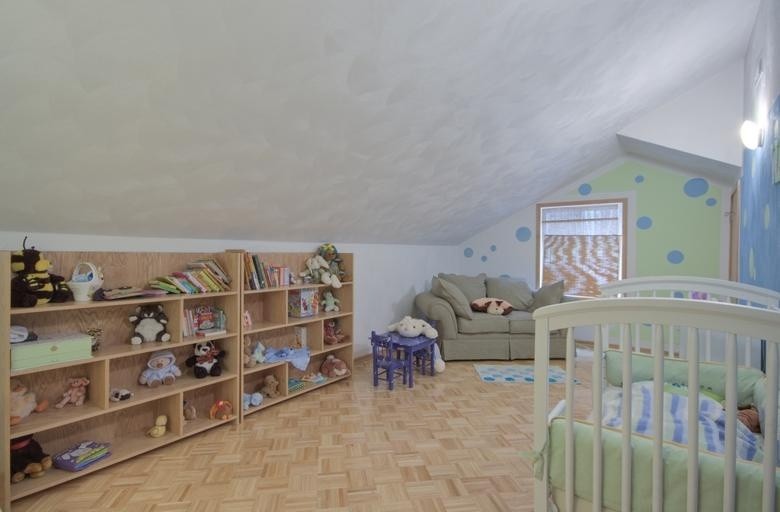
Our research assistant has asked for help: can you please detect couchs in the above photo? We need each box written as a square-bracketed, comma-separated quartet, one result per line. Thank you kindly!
[415, 289, 577, 362]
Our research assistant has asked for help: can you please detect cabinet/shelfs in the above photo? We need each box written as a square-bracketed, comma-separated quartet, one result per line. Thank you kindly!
[240, 252, 355, 421]
[0, 252, 239, 511]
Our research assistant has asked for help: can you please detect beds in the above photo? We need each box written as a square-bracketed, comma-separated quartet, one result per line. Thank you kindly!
[531, 275, 780, 512]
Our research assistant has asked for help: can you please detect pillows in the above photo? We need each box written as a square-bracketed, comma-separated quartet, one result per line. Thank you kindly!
[438, 272, 487, 305]
[485, 277, 535, 311]
[528, 279, 564, 313]
[432, 275, 473, 320]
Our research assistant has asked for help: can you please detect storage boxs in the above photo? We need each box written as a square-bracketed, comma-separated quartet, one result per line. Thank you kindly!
[288, 287, 319, 318]
[10, 330, 95, 372]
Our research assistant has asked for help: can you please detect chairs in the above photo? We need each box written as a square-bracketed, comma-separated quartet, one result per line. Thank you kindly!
[397, 319, 438, 375]
[371, 331, 408, 390]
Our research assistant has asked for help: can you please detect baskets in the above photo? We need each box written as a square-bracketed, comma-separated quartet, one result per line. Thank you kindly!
[65, 262, 104, 302]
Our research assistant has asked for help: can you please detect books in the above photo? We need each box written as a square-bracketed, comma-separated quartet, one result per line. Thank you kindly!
[228, 249, 289, 289]
[103, 258, 230, 299]
[295, 326, 307, 349]
[184, 304, 227, 337]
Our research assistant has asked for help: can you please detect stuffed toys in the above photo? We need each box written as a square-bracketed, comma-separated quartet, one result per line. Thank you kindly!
[321, 292, 339, 312]
[471, 297, 514, 316]
[206, 399, 233, 422]
[321, 354, 351, 377]
[185, 341, 225, 378]
[244, 336, 256, 367]
[261, 377, 280, 394]
[324, 320, 346, 343]
[127, 304, 171, 344]
[315, 242, 344, 289]
[244, 393, 264, 410]
[137, 352, 181, 387]
[254, 341, 265, 362]
[10, 435, 51, 483]
[12, 236, 67, 308]
[149, 416, 167, 438]
[299, 255, 328, 282]
[387, 317, 446, 373]
[9, 378, 48, 426]
[54, 375, 89, 408]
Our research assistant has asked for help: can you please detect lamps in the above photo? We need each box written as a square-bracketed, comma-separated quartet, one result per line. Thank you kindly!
[740, 120, 765, 150]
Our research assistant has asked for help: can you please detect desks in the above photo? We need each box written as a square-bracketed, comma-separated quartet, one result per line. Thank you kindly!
[368, 330, 438, 388]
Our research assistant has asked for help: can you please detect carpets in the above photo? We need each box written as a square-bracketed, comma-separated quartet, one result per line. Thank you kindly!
[473, 364, 581, 385]
